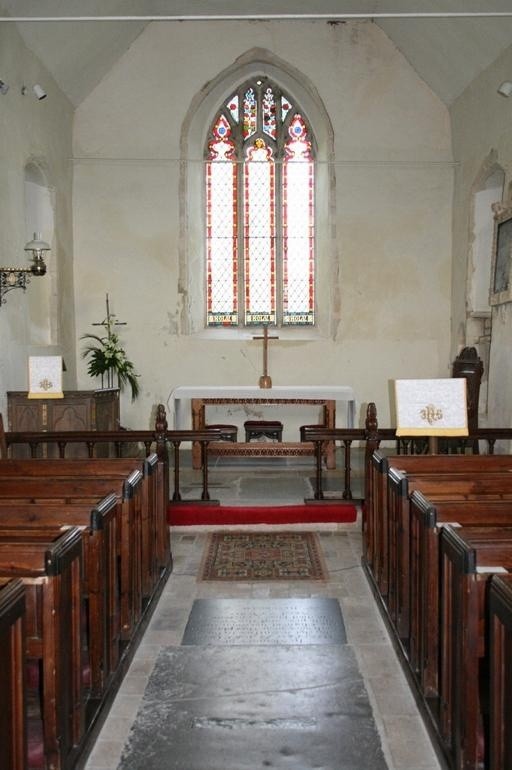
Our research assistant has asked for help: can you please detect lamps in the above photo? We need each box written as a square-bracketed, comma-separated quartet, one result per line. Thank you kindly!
[0, 231, 51, 308]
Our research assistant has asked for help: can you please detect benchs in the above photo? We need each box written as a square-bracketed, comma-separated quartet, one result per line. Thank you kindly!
[1, 453, 173, 766]
[358, 447, 512, 770]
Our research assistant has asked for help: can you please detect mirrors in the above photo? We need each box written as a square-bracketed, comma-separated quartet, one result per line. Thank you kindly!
[465, 164, 506, 318]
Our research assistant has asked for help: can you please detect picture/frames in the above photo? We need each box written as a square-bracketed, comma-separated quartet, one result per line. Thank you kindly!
[486, 206, 512, 309]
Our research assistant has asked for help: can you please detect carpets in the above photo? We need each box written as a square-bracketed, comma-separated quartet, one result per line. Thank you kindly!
[196, 530, 331, 585]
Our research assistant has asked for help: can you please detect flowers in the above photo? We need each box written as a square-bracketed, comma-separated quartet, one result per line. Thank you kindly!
[77, 319, 142, 405]
